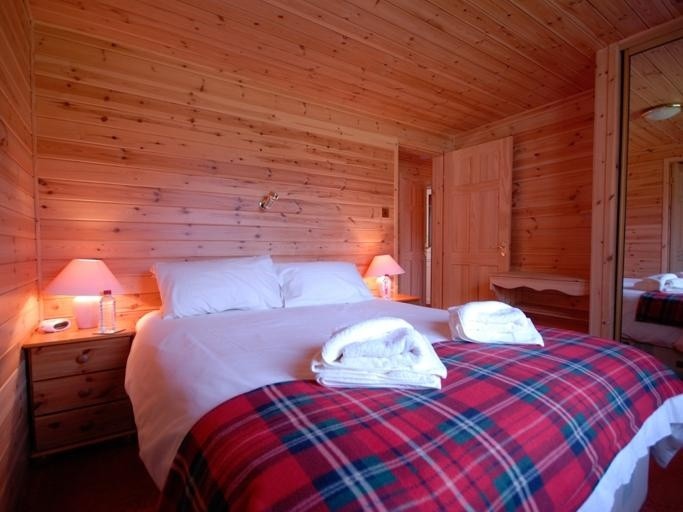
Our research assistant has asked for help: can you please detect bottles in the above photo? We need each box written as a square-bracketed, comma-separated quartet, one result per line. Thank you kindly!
[99, 289, 116, 333]
[383, 274, 391, 303]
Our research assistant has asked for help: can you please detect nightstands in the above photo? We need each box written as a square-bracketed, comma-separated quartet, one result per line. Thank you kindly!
[359, 253, 406, 298]
[24, 324, 140, 463]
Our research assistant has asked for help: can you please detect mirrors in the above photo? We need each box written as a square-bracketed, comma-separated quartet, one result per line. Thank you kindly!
[610, 31, 682, 377]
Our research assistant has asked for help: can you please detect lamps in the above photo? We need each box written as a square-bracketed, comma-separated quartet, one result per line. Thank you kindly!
[639, 102, 681, 122]
[38, 255, 130, 329]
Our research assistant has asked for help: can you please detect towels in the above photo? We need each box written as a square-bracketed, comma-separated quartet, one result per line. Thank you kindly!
[634, 270, 681, 295]
[302, 298, 546, 392]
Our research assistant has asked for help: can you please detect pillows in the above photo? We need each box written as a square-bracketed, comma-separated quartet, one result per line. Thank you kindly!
[150, 253, 375, 320]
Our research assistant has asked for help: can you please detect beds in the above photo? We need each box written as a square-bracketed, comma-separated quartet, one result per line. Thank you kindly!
[135, 298, 682, 511]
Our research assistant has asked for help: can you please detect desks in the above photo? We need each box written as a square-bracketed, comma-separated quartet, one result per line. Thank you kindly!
[488, 271, 590, 332]
